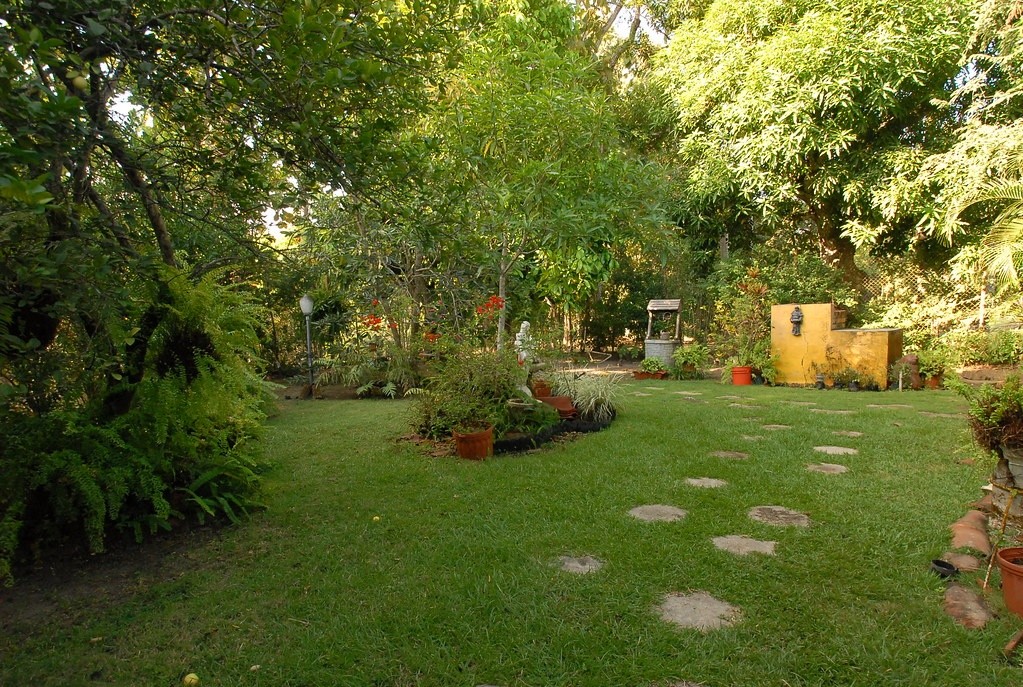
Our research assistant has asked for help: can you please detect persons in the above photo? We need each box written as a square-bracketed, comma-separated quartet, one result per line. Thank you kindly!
[790, 306, 803, 336]
[515, 322, 535, 396]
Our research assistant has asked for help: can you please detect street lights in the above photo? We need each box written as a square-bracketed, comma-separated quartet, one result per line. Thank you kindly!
[299, 294, 314, 384]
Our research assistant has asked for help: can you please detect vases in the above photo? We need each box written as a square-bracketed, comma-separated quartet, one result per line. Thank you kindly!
[997, 547, 1023, 620]
[731, 366, 752, 385]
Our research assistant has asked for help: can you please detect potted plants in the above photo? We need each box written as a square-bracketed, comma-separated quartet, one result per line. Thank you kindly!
[671, 342, 710, 372]
[433, 403, 499, 461]
[632, 356, 666, 380]
[968, 371, 1023, 517]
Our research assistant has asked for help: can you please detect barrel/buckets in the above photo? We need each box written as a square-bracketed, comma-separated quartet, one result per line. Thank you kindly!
[732, 367, 751, 384]
